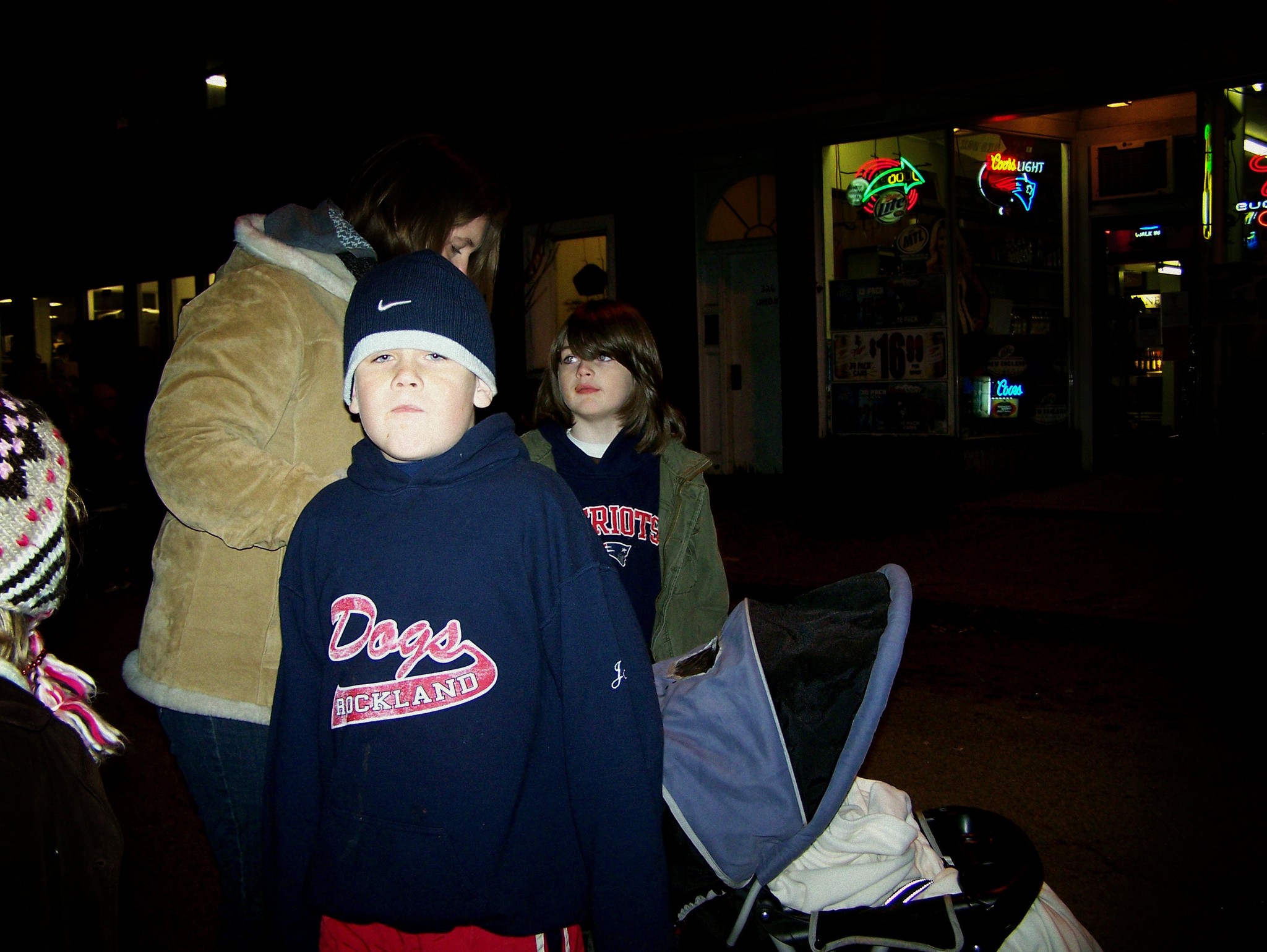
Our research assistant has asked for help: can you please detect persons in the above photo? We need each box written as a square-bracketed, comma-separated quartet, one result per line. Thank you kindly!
[517, 305, 728, 659]
[0, 391, 132, 952]
[925, 217, 990, 335]
[120, 134, 502, 913]
[260, 250, 670, 952]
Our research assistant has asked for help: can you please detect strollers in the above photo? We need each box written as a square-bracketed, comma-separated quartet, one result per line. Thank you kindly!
[651, 563, 1102, 952]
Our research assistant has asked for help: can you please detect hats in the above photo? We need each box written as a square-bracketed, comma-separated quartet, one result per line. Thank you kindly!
[342, 247, 500, 403]
[0, 384, 73, 622]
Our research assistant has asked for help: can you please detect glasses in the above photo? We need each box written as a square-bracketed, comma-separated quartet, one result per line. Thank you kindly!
[54, 490, 87, 539]
